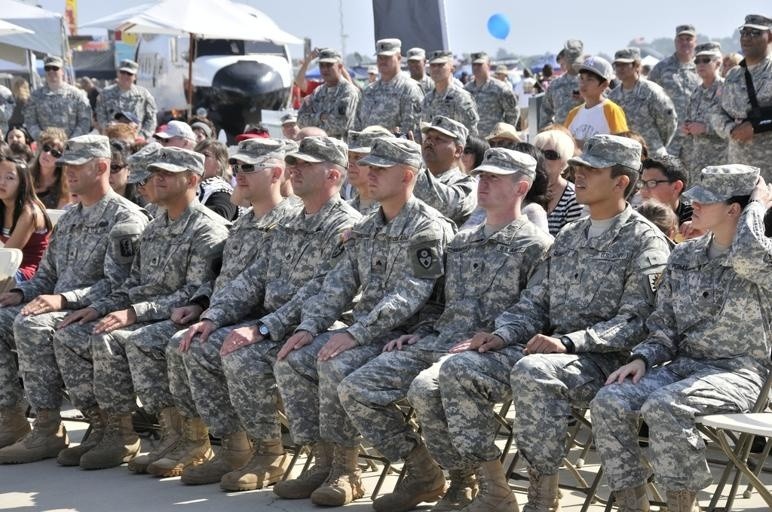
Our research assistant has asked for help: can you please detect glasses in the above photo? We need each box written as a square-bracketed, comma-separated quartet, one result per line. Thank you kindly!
[232, 164, 273, 174]
[45, 66, 59, 71]
[536, 148, 561, 161]
[694, 57, 711, 64]
[43, 145, 61, 157]
[464, 147, 474, 155]
[635, 179, 673, 188]
[489, 139, 508, 148]
[739, 30, 761, 37]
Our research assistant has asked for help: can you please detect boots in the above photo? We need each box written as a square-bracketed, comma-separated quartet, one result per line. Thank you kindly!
[310, 446, 366, 506]
[272, 440, 335, 500]
[461, 458, 519, 512]
[181, 431, 253, 484]
[0, 408, 68, 464]
[80, 412, 141, 468]
[521, 466, 559, 512]
[665, 490, 699, 512]
[615, 486, 651, 512]
[220, 438, 292, 491]
[56, 414, 107, 467]
[431, 466, 479, 512]
[372, 446, 445, 512]
[0, 410, 32, 448]
[147, 416, 215, 478]
[127, 407, 179, 472]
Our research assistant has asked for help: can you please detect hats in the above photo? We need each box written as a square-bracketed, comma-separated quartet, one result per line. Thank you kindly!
[356, 138, 422, 168]
[470, 146, 536, 181]
[315, 49, 342, 63]
[680, 163, 761, 205]
[234, 127, 270, 144]
[374, 39, 488, 65]
[228, 138, 285, 169]
[347, 125, 396, 154]
[284, 136, 349, 169]
[494, 65, 507, 75]
[565, 38, 641, 79]
[44, 55, 62, 68]
[695, 44, 721, 56]
[119, 60, 138, 74]
[54, 122, 212, 177]
[421, 114, 470, 149]
[738, 14, 772, 31]
[676, 25, 695, 38]
[280, 114, 298, 126]
[566, 133, 641, 171]
[483, 121, 521, 143]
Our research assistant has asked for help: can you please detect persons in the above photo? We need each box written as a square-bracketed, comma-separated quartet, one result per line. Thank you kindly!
[1, 0, 770, 510]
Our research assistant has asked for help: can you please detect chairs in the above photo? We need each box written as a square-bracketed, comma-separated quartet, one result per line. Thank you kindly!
[0, 210, 772, 511]
